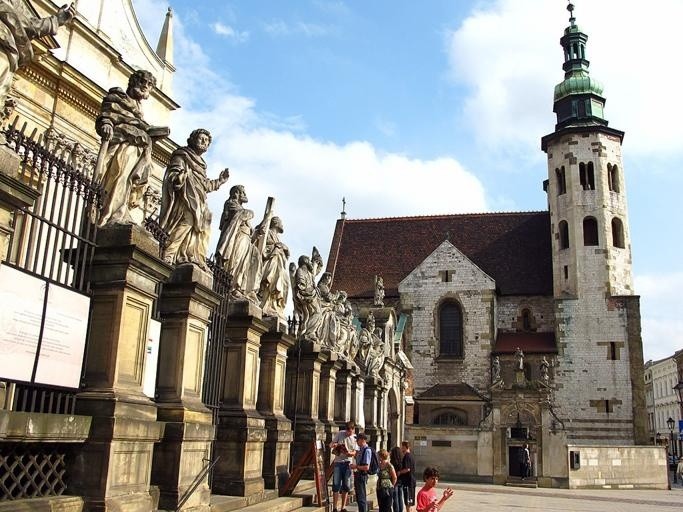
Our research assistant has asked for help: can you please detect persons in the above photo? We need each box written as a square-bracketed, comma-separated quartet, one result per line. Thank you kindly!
[669, 453, 683, 487]
[513, 347, 524, 373]
[349, 431, 372, 512]
[215, 184, 264, 303]
[251, 215, 291, 319]
[328, 420, 359, 512]
[538, 355, 549, 381]
[389, 445, 404, 512]
[157, 129, 231, 269]
[291, 253, 387, 378]
[492, 356, 501, 378]
[93, 69, 171, 226]
[375, 449, 398, 512]
[396, 439, 416, 512]
[519, 442, 531, 480]
[417, 466, 453, 512]
[0, 1, 77, 121]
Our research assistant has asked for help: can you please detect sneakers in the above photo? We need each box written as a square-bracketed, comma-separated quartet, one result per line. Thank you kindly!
[341, 508, 347, 512]
[333, 508, 337, 512]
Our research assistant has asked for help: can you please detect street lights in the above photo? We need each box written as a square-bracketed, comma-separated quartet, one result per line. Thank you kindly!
[656, 431, 661, 445]
[665, 416, 677, 484]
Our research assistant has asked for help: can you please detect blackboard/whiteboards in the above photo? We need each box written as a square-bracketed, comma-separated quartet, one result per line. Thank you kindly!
[311, 438, 330, 501]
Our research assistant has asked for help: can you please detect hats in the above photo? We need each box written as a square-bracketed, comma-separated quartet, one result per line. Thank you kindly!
[354, 433, 368, 440]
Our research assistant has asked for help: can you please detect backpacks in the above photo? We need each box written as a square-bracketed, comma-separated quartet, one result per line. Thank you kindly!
[361, 447, 379, 475]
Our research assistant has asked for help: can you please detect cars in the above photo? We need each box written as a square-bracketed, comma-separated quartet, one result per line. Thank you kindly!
[668, 455, 678, 467]
